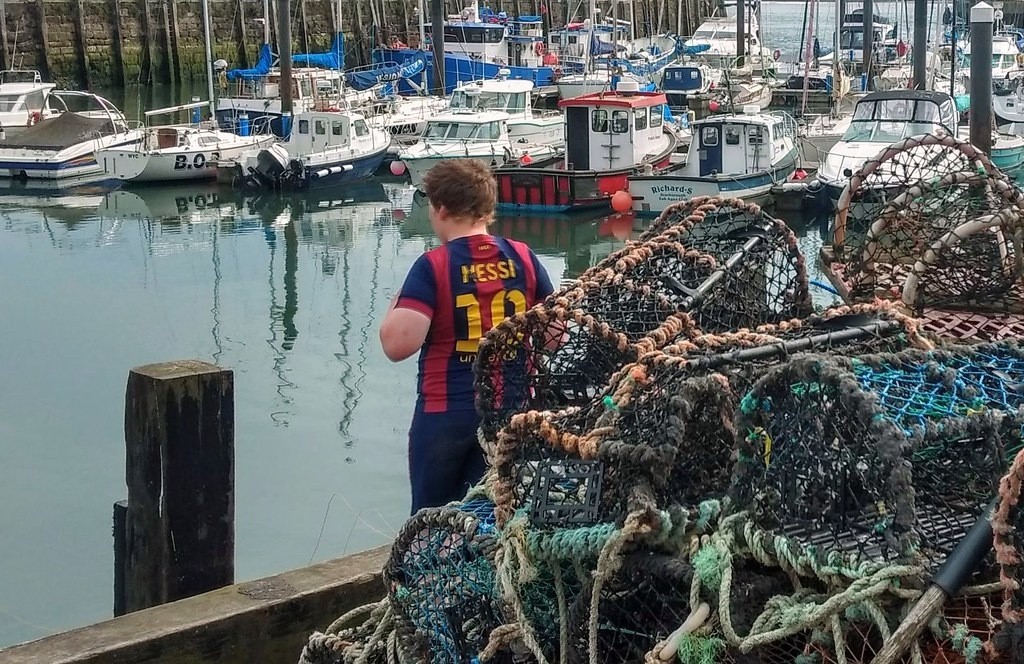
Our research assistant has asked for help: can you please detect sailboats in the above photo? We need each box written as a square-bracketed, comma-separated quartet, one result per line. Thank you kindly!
[1, 0, 1023, 220]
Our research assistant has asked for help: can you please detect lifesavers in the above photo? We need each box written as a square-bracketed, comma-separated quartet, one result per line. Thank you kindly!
[536, 41, 544, 56]
[27, 111, 40, 129]
[773, 50, 781, 61]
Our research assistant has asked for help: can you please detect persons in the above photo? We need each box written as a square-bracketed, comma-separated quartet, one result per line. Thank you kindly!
[379, 157, 566, 515]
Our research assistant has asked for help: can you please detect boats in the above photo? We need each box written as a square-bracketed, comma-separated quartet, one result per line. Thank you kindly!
[398, 105, 558, 192]
[444, 69, 566, 147]
[190, 175, 391, 226]
[1, 178, 127, 234]
[811, 85, 1024, 219]
[486, 208, 630, 257]
[1, 67, 124, 132]
[207, 104, 393, 194]
[823, 221, 923, 257]
[628, 105, 801, 216]
[1, 89, 146, 180]
[491, 80, 678, 213]
